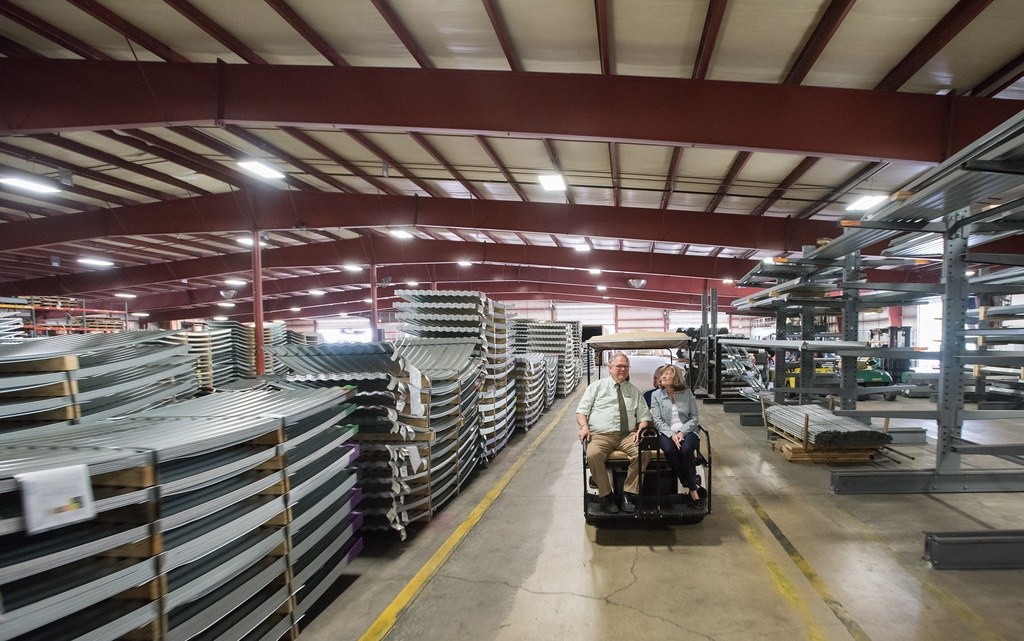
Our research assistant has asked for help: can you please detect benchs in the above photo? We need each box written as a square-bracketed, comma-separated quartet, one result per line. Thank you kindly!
[585, 437, 704, 472]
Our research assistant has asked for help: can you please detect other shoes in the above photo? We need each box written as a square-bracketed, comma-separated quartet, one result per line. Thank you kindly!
[694, 482, 708, 499]
[687, 492, 705, 509]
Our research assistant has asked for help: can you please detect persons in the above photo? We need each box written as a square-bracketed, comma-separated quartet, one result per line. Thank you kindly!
[650, 364, 708, 509]
[642, 366, 665, 409]
[576, 352, 654, 513]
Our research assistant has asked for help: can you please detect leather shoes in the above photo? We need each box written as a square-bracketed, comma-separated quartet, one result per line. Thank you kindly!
[603, 492, 619, 514]
[620, 492, 636, 513]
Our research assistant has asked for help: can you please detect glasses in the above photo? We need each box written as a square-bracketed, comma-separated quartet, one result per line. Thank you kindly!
[611, 364, 632, 370]
[661, 371, 674, 376]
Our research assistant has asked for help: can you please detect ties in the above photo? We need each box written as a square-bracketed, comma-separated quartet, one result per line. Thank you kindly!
[614, 384, 630, 438]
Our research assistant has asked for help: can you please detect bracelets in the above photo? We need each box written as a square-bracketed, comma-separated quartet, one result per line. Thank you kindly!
[579, 424, 588, 430]
